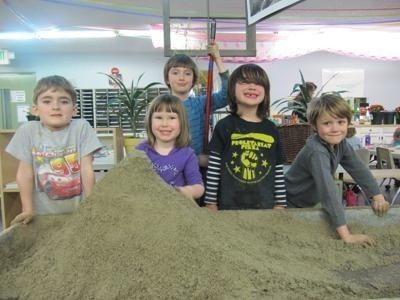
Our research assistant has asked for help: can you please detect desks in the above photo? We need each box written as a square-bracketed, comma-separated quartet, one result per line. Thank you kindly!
[339, 168, 400, 208]
[0, 126, 123, 230]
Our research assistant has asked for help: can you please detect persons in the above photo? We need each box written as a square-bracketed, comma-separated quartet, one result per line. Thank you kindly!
[163, 40, 231, 207]
[284, 94, 389, 247]
[134, 95, 205, 201]
[5, 75, 101, 225]
[202, 63, 286, 210]
[289, 83, 314, 122]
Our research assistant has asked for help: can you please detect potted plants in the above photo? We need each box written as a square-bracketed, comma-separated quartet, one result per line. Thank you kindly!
[369, 105, 383, 125]
[101, 69, 163, 157]
[396, 105, 400, 124]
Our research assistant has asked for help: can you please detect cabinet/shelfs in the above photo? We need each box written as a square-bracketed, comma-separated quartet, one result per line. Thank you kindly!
[1, 72, 36, 130]
[73, 89, 171, 127]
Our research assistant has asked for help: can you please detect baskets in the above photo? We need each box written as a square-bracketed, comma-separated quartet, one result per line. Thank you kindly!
[276, 124, 310, 163]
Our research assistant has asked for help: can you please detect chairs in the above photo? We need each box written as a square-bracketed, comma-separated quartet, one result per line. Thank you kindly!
[377, 147, 400, 190]
